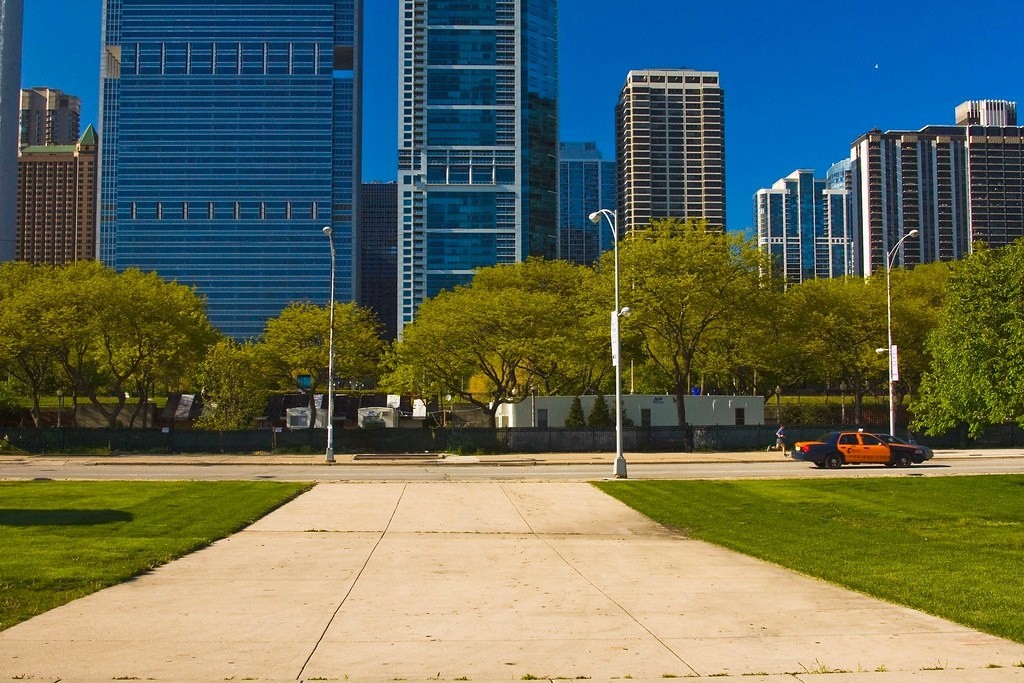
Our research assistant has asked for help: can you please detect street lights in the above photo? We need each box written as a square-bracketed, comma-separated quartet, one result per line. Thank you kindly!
[322, 225, 336, 460]
[589, 209, 630, 480]
[875, 230, 921, 436]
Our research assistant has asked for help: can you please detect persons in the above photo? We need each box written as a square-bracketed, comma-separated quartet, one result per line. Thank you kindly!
[684, 422, 693, 453]
[767, 424, 789, 458]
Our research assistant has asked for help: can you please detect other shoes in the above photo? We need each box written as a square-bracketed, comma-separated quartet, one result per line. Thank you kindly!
[784, 454, 788, 457]
[767, 445, 771, 451]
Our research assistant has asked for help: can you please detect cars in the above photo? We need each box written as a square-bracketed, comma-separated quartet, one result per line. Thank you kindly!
[791, 431, 934, 469]
